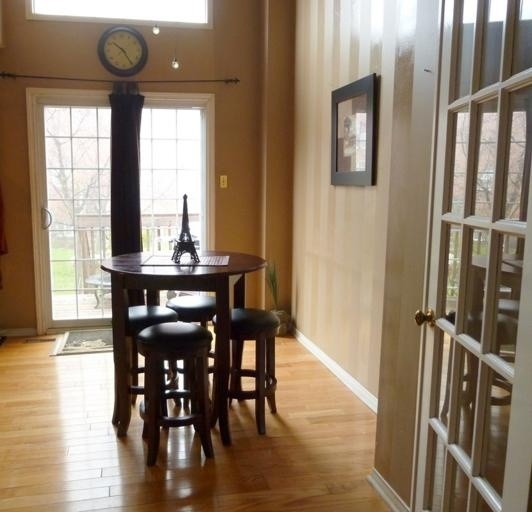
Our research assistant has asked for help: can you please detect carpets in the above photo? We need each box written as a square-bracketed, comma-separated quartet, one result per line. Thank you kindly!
[50, 329, 113, 355]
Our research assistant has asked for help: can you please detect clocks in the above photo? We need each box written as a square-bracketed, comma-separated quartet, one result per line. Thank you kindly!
[97, 25, 148, 76]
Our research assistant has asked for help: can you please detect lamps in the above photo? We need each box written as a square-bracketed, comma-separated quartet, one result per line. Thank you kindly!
[171, 57, 180, 70]
[151, 23, 160, 36]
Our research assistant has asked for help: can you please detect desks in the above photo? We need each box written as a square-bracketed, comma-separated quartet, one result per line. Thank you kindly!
[100, 250, 267, 446]
[471, 253, 522, 391]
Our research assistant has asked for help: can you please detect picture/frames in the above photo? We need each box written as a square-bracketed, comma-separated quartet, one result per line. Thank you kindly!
[330, 73, 375, 186]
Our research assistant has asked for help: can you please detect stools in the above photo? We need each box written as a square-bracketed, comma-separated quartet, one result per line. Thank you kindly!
[166, 295, 217, 417]
[462, 312, 508, 414]
[136, 321, 214, 466]
[125, 304, 180, 406]
[212, 308, 281, 436]
